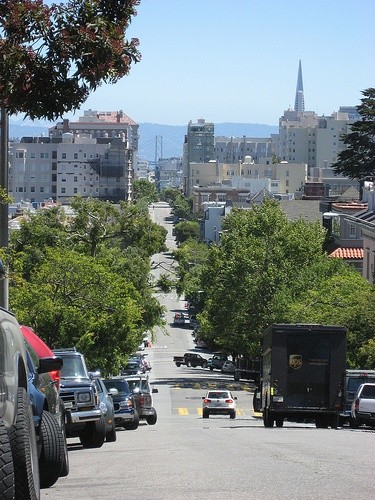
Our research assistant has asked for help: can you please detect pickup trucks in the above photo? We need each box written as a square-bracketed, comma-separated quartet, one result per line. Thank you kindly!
[173, 353, 207, 368]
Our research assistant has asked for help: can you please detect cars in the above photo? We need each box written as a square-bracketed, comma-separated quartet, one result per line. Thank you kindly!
[121, 361, 144, 376]
[93, 377, 118, 442]
[221, 360, 237, 373]
[208, 357, 213, 366]
[173, 313, 185, 326]
[190, 315, 210, 349]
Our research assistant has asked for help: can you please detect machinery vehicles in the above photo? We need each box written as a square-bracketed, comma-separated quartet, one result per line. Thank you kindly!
[234, 355, 263, 384]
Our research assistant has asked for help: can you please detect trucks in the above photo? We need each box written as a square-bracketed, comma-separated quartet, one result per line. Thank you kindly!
[253, 323, 349, 428]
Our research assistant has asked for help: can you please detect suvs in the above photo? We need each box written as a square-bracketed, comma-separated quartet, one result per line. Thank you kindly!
[125, 378, 159, 425]
[0, 305, 70, 500]
[51, 347, 106, 449]
[343, 369, 375, 428]
[202, 389, 238, 419]
[100, 378, 140, 430]
[210, 353, 228, 371]
